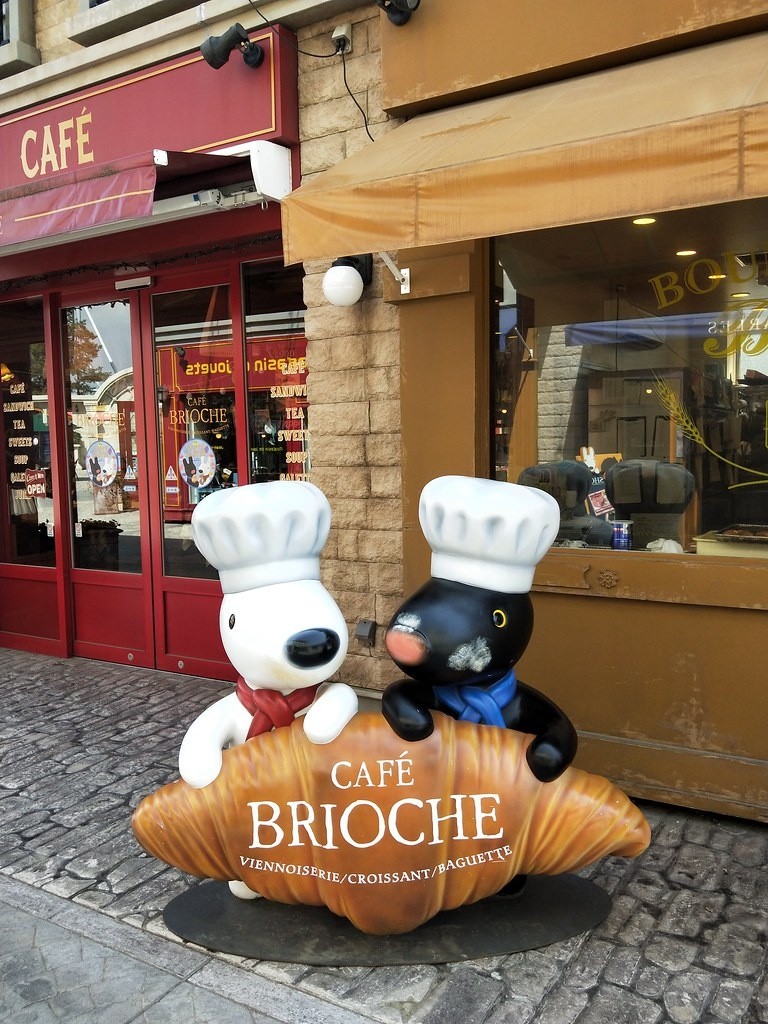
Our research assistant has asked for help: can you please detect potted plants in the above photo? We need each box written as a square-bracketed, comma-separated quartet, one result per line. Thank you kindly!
[73, 518, 124, 571]
[82, 475, 130, 515]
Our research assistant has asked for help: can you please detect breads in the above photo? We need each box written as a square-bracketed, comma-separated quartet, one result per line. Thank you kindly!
[130, 710, 651, 936]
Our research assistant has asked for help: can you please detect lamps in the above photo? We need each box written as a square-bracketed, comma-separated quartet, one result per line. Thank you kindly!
[201, 23, 264, 70]
[322, 253, 372, 307]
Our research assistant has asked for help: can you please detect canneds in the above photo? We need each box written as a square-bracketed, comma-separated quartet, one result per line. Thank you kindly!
[610, 520, 634, 550]
